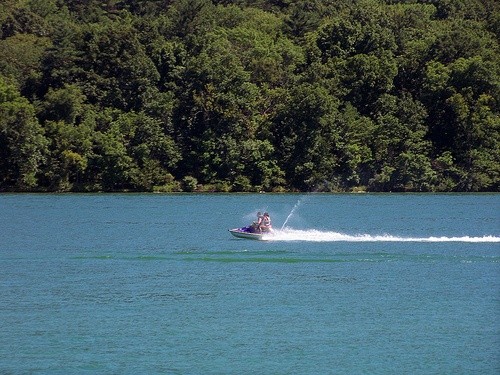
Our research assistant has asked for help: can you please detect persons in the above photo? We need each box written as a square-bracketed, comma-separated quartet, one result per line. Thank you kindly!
[250, 212, 264, 232]
[258, 212, 271, 233]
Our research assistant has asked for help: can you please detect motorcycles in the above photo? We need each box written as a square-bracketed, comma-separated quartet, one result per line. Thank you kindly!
[228, 220, 274, 240]
